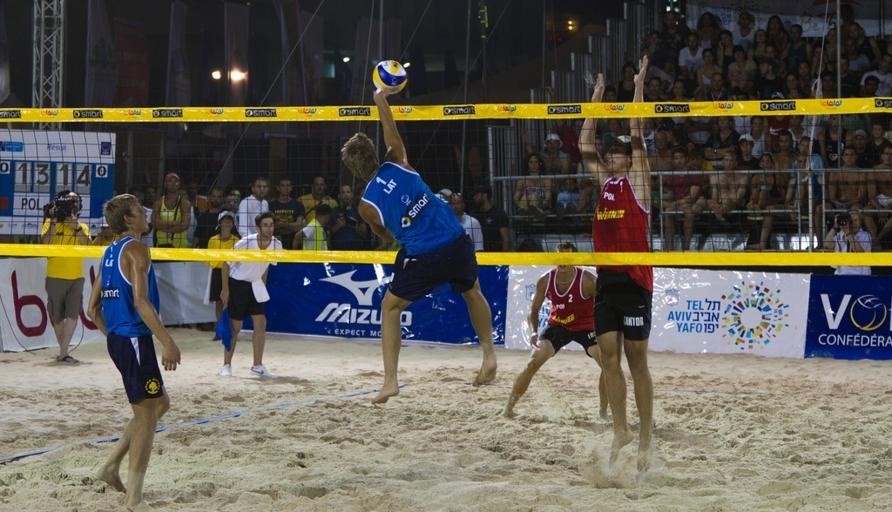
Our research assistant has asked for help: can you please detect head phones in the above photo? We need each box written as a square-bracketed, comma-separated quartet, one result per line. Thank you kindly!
[78, 193, 84, 212]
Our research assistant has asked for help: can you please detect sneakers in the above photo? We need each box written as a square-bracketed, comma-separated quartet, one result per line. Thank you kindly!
[220, 364, 232, 376]
[249, 365, 276, 378]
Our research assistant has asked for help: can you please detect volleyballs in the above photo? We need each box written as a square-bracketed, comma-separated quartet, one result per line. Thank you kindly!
[372, 60, 408, 94]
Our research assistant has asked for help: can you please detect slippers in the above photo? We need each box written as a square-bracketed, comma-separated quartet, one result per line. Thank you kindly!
[58, 355, 73, 363]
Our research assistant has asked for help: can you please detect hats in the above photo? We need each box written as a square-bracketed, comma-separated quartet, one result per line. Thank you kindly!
[438, 189, 452, 197]
[738, 134, 754, 142]
[471, 186, 488, 196]
[543, 133, 563, 148]
[604, 133, 632, 144]
[166, 173, 179, 181]
[854, 128, 867, 138]
[218, 211, 236, 223]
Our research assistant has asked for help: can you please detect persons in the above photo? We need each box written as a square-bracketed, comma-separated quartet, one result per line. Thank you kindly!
[38, 172, 511, 263]
[501, 242, 608, 418]
[40, 190, 92, 365]
[221, 212, 284, 375]
[87, 193, 181, 512]
[206, 210, 241, 341]
[341, 89, 498, 404]
[511, 6, 892, 252]
[577, 55, 653, 471]
